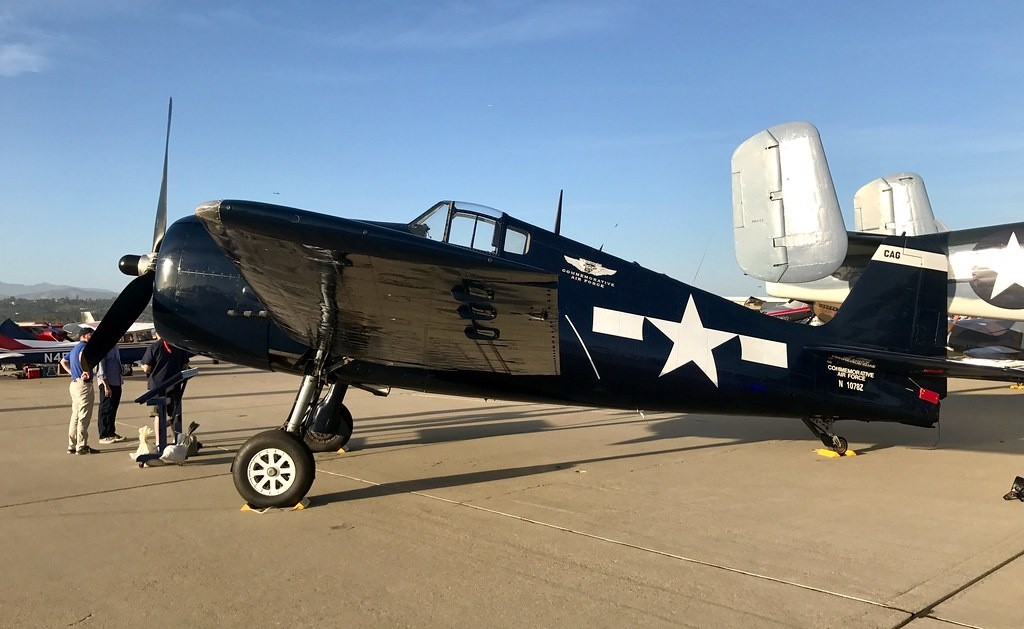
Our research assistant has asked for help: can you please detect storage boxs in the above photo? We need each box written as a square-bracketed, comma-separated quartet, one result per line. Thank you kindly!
[28, 368, 40, 379]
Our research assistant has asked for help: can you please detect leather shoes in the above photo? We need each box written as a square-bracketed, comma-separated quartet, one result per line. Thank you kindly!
[73, 379, 93, 384]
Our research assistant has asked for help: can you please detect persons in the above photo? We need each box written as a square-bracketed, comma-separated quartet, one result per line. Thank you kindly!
[97, 343, 127, 443]
[60, 326, 100, 455]
[140, 327, 190, 452]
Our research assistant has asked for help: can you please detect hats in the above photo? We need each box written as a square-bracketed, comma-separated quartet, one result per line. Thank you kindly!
[78, 327, 95, 338]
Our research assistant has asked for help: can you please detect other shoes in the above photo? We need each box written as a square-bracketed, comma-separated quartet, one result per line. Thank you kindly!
[109, 435, 127, 442]
[75, 446, 102, 455]
[66, 447, 75, 454]
[99, 437, 114, 445]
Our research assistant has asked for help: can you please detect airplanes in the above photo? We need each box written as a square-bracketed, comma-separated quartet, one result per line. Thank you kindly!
[77, 96, 1024, 508]
[0, 313, 160, 376]
